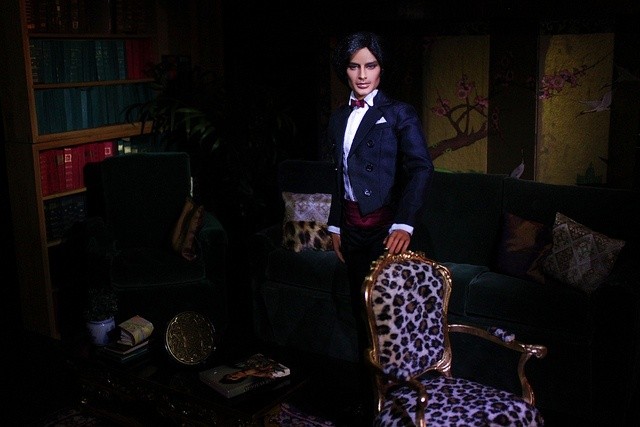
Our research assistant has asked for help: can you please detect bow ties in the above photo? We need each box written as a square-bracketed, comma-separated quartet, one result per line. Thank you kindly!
[351, 99, 364, 108]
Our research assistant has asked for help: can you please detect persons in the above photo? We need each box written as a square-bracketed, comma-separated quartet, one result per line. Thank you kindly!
[325, 32, 434, 268]
[217, 355, 290, 385]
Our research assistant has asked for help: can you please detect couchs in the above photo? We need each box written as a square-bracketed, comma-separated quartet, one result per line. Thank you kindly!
[222, 157, 627, 423]
[76, 151, 228, 331]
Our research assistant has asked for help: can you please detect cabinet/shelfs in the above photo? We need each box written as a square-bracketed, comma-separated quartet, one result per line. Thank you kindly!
[3, 2, 205, 342]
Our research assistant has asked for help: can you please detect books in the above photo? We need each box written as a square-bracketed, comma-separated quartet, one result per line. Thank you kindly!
[197, 353, 292, 398]
[117, 315, 153, 346]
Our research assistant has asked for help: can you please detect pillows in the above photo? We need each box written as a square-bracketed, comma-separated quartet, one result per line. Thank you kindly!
[284, 190, 332, 222]
[503, 212, 544, 279]
[528, 209, 627, 300]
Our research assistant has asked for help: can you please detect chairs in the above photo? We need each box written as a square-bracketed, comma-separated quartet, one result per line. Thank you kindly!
[365, 237, 545, 427]
